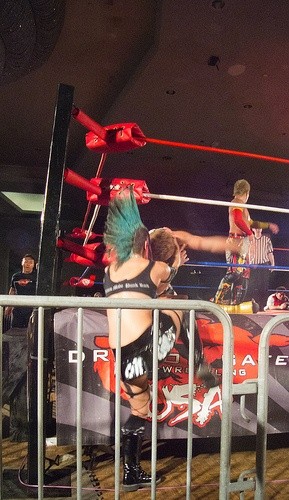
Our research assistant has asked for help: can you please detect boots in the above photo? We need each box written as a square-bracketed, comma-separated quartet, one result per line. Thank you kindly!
[181, 313, 204, 368]
[121, 414, 161, 491]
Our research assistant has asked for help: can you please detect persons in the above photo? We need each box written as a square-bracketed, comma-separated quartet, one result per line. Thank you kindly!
[214, 179, 279, 305]
[103, 226, 221, 492]
[239, 222, 275, 312]
[263, 287, 289, 309]
[5, 254, 38, 329]
